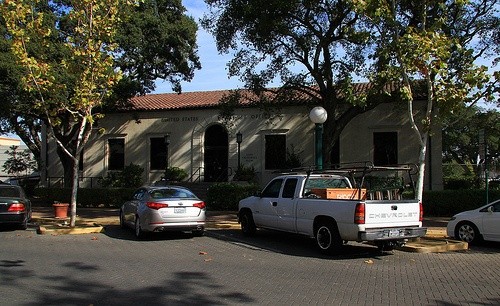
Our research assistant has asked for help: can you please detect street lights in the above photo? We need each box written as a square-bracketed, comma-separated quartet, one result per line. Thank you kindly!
[310, 106, 328, 169]
[236, 130, 242, 169]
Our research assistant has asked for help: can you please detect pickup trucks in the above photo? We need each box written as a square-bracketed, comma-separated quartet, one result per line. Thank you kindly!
[237, 167, 428, 255]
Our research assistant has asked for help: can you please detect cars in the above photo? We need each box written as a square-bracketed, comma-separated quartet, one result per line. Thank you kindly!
[447, 199, 500, 244]
[119, 186, 206, 241]
[0, 185, 32, 230]
